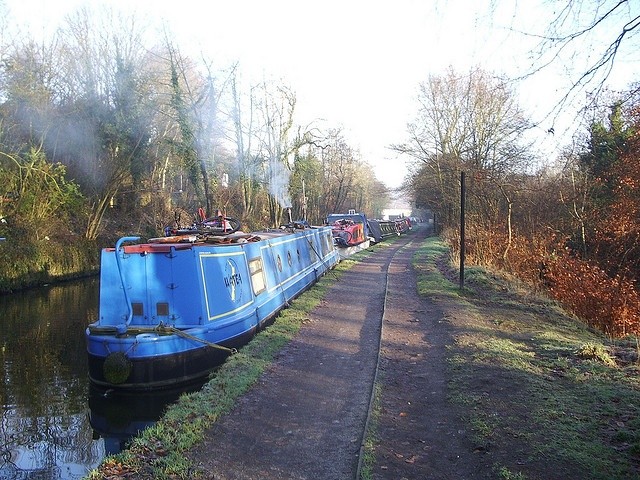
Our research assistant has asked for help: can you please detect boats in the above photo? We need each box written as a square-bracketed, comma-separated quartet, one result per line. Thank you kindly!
[392, 215, 411, 234]
[322, 208, 370, 261]
[81, 207, 341, 400]
[366, 219, 398, 246]
